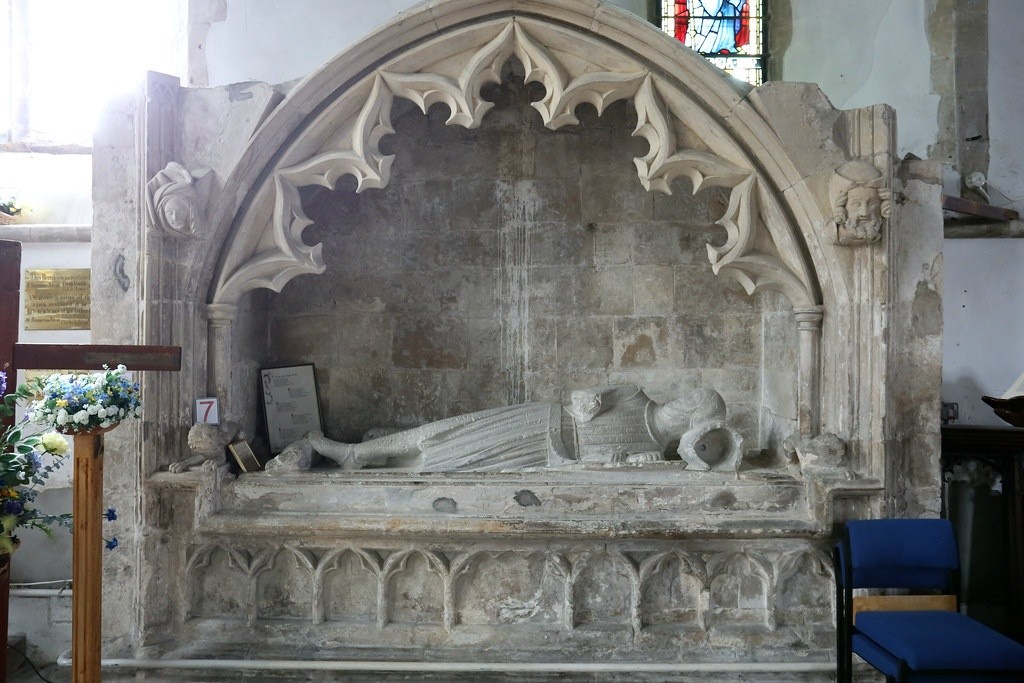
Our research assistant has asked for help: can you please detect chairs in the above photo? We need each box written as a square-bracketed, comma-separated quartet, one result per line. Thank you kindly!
[834, 519, 1024, 683]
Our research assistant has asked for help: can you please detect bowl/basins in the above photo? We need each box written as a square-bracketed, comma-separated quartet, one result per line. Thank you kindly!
[980, 394, 1024, 408]
[992, 408, 1024, 427]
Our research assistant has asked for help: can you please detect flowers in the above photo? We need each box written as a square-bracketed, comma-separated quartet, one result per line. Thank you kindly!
[0, 364, 143, 558]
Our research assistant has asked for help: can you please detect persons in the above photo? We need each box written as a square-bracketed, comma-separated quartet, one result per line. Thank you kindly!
[310, 383, 724, 474]
[153, 181, 195, 234]
[830, 161, 885, 237]
